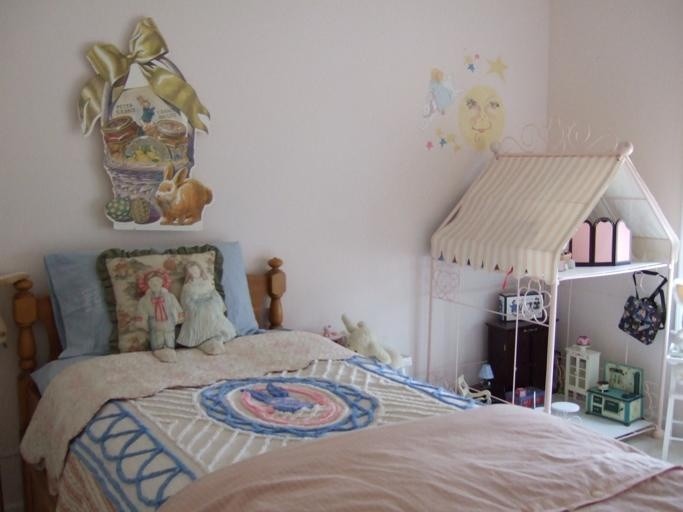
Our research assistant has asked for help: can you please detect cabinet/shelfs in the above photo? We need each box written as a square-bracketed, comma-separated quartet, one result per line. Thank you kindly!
[564, 345, 601, 403]
[486, 315, 560, 397]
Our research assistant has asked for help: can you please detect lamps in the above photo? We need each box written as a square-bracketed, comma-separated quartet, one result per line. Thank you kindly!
[479, 364, 494, 389]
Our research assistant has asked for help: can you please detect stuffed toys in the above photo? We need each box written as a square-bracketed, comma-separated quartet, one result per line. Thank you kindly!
[340, 313, 402, 369]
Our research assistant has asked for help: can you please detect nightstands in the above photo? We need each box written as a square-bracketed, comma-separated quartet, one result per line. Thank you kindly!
[399, 355, 411, 374]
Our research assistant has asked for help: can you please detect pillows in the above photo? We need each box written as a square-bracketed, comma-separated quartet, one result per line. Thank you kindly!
[97, 245, 225, 352]
[45, 241, 262, 359]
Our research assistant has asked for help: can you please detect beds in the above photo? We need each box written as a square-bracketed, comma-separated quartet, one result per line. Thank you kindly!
[15, 257, 683, 512]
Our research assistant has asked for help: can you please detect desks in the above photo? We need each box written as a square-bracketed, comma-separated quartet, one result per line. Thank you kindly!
[662, 354, 683, 462]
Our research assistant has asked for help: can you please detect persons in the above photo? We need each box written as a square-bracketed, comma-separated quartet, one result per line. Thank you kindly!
[135, 270, 185, 363]
[175, 262, 237, 355]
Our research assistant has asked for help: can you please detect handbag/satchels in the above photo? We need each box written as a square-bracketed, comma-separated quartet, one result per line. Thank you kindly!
[616, 270, 668, 346]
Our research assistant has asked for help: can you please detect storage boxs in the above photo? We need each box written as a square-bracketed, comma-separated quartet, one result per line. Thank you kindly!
[499, 293, 543, 321]
